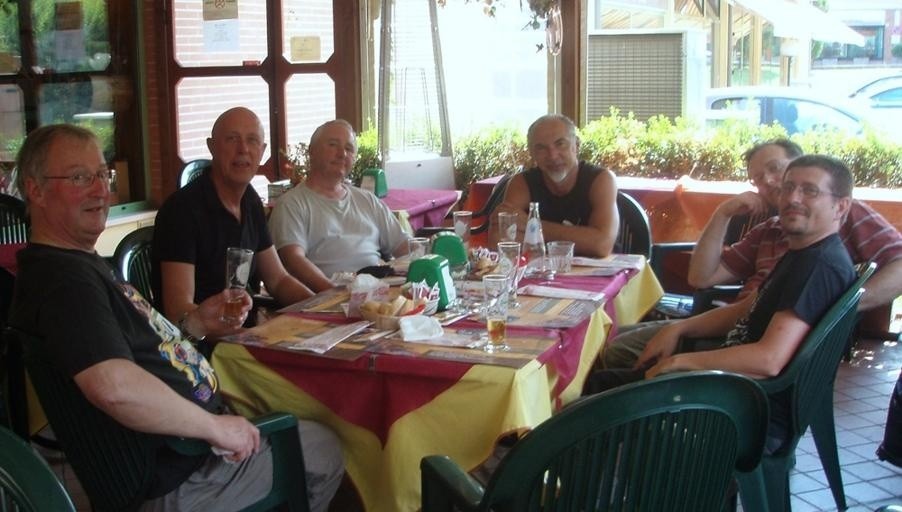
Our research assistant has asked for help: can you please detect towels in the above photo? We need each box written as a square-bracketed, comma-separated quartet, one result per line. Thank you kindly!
[391, 305, 445, 344]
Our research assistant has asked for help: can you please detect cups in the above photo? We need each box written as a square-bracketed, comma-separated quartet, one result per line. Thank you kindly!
[481, 274, 509, 353]
[408, 209, 575, 316]
[222, 245, 255, 323]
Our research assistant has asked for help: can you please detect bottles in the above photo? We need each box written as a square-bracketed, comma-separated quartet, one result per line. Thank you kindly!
[520, 202, 547, 279]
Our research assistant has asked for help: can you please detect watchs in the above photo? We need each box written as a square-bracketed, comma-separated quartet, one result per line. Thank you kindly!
[177, 313, 206, 343]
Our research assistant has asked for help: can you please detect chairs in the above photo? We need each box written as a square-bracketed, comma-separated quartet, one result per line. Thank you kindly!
[610, 180, 653, 257]
[641, 241, 741, 351]
[114, 222, 279, 331]
[1, 406, 313, 512]
[419, 364, 771, 510]
[639, 259, 883, 511]
[414, 162, 525, 240]
[179, 159, 214, 192]
[1, 194, 32, 245]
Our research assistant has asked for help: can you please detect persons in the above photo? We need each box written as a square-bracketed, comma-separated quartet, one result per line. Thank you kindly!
[488, 114, 619, 257]
[269, 120, 422, 293]
[592, 155, 856, 462]
[14, 124, 344, 512]
[603, 139, 902, 373]
[152, 107, 315, 346]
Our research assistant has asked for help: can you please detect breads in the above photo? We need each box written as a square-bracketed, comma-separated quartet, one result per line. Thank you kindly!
[365, 295, 428, 316]
[470, 257, 501, 279]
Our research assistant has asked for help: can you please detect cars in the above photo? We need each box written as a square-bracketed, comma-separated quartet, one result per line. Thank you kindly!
[843, 72, 902, 137]
[703, 84, 883, 142]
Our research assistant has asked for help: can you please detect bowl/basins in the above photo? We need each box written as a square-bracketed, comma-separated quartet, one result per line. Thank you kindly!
[356, 304, 425, 327]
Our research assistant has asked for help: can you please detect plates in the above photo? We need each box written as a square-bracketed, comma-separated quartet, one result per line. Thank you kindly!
[378, 274, 408, 287]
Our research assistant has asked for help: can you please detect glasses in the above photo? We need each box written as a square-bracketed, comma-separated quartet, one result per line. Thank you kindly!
[748, 156, 787, 186]
[35, 169, 118, 188]
[779, 182, 842, 202]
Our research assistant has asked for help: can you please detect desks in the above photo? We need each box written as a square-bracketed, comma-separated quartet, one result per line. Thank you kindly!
[378, 183, 464, 230]
[465, 167, 902, 343]
